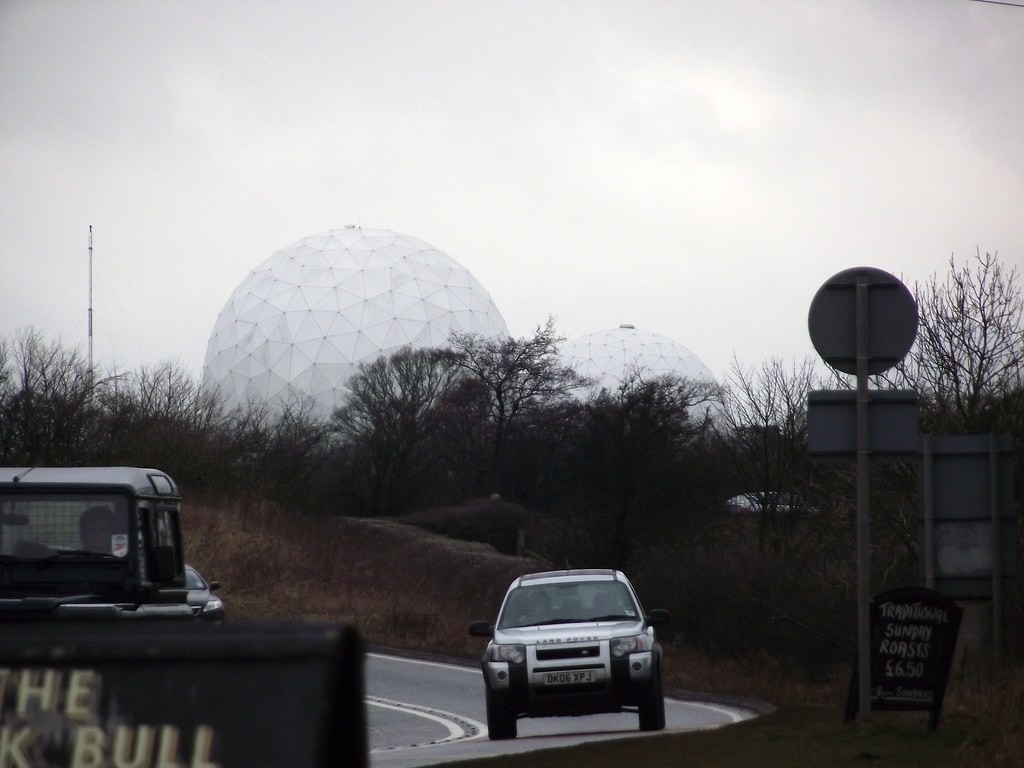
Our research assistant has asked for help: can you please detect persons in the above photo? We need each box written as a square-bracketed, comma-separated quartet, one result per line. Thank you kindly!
[80, 507, 118, 554]
[519, 591, 553, 622]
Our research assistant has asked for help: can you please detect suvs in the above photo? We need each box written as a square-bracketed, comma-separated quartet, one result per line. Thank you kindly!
[468, 568, 674, 740]
[0, 467, 196, 627]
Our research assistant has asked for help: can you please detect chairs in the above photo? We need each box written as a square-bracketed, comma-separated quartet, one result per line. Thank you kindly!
[593, 590, 622, 617]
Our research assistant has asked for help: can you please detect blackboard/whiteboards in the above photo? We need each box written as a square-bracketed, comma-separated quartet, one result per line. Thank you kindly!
[849, 587, 963, 712]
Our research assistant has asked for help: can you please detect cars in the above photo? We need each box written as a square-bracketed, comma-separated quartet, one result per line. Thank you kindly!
[182, 564, 226, 623]
[728, 490, 825, 517]
